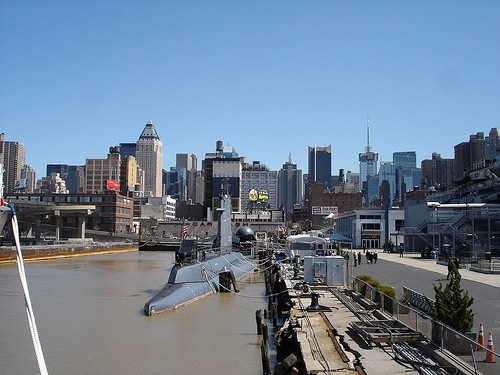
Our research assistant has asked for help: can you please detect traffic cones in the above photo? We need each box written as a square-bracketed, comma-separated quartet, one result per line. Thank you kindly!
[483, 331, 496, 363]
[477, 322, 486, 351]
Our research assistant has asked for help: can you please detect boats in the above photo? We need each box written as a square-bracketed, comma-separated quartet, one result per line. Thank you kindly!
[144, 193, 257, 316]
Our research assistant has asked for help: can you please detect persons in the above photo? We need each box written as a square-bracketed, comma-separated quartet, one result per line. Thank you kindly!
[325, 239, 405, 268]
[446, 254, 460, 280]
